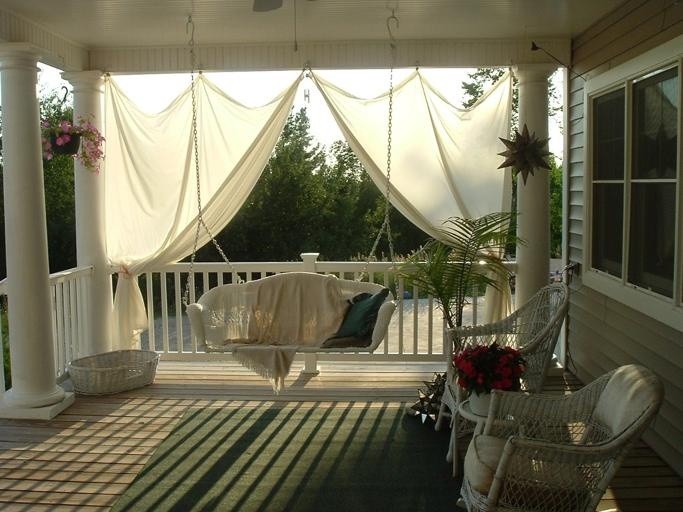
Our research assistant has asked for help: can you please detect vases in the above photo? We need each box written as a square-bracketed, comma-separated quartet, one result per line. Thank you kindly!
[48, 129, 79, 154]
[453, 342, 526, 417]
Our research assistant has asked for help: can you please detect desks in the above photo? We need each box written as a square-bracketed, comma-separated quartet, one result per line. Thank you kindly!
[457, 389, 535, 476]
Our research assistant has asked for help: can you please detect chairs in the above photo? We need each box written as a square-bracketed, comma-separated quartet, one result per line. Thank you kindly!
[454, 363, 665, 512]
[433, 281, 568, 462]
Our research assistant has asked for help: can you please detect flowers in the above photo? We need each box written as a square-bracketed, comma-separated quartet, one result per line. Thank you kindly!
[32, 97, 107, 176]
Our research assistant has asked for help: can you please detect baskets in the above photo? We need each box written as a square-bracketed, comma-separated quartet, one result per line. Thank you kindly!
[68, 348, 161, 396]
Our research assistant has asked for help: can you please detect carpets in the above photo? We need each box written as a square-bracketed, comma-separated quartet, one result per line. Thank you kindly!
[108, 405, 465, 512]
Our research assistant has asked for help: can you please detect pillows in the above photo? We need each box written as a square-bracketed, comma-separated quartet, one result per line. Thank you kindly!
[321, 288, 391, 348]
